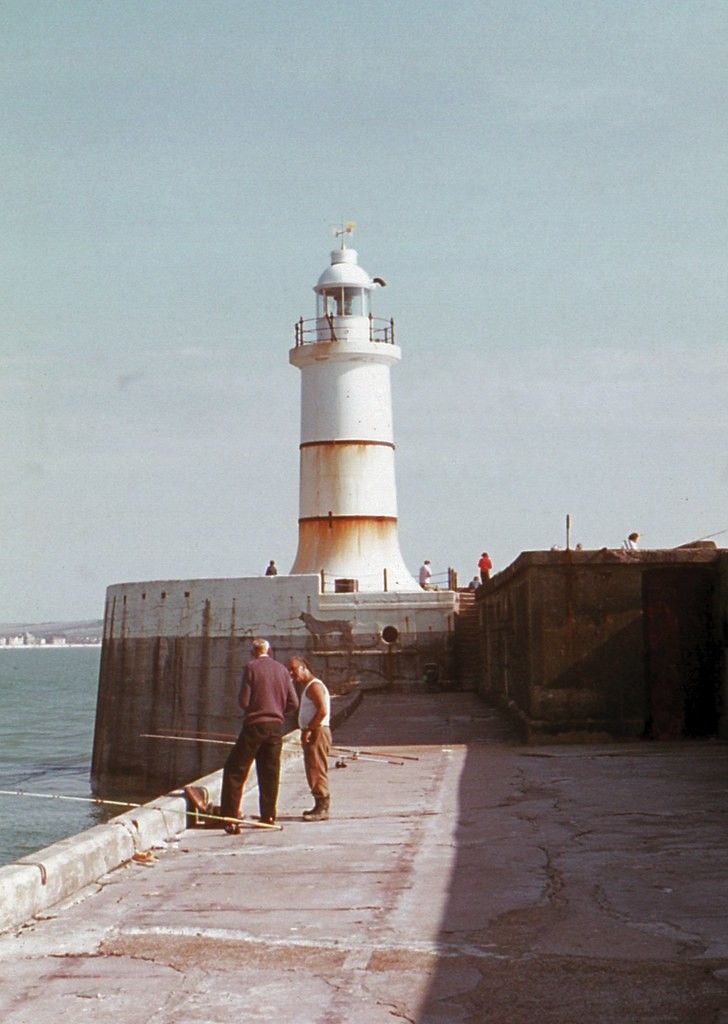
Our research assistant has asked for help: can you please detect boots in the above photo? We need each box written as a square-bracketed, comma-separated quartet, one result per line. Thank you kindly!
[302, 795, 331, 821]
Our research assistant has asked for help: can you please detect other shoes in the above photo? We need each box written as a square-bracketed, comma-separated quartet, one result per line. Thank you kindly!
[258, 817, 274, 828]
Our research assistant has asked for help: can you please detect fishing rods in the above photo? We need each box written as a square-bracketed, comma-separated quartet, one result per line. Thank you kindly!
[137, 733, 405, 768]
[156, 727, 420, 762]
[0, 790, 285, 832]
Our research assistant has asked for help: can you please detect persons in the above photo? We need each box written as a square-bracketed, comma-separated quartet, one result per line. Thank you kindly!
[478, 552, 492, 584]
[469, 575, 480, 589]
[621, 533, 638, 550]
[266, 560, 278, 576]
[576, 543, 583, 551]
[221, 637, 299, 828]
[288, 656, 334, 821]
[419, 560, 432, 589]
